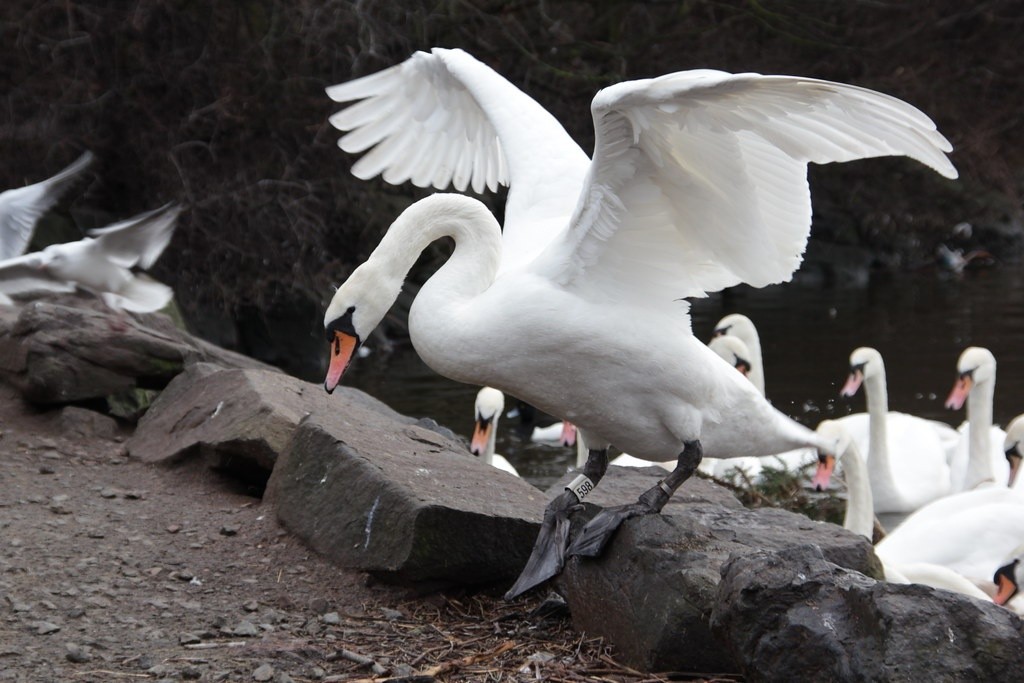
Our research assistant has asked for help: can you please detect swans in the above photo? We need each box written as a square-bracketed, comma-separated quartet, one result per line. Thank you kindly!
[470, 311, 1024, 611]
[0, 151, 183, 333]
[322, 46, 958, 625]
